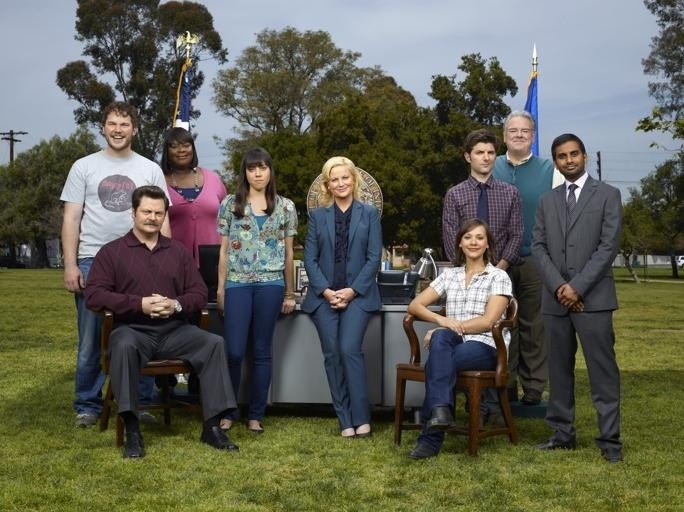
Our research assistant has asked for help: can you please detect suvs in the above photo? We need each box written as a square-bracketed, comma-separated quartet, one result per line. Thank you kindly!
[677, 255, 684, 268]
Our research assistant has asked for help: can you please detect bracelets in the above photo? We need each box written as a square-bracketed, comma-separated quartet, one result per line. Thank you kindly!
[283, 292, 295, 298]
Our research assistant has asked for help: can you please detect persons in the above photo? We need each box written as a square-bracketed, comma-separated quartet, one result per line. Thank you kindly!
[441, 128, 523, 427]
[83, 186, 241, 458]
[157, 127, 229, 304]
[406, 219, 513, 459]
[485, 110, 557, 404]
[214, 147, 298, 433]
[299, 155, 383, 442]
[59, 102, 173, 431]
[531, 132, 626, 462]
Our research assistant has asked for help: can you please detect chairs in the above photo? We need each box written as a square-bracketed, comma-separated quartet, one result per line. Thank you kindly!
[393, 297, 520, 458]
[98, 293, 211, 447]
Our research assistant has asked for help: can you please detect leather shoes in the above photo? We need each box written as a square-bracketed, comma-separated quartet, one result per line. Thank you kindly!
[341, 389, 622, 462]
[75, 386, 264, 459]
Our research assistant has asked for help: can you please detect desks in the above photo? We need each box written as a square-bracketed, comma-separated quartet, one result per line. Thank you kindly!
[177, 300, 497, 424]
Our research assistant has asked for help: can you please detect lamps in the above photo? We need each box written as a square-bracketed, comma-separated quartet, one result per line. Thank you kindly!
[412, 247, 439, 285]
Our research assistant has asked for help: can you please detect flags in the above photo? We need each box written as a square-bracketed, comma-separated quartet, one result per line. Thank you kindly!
[517, 68, 540, 157]
[168, 55, 198, 131]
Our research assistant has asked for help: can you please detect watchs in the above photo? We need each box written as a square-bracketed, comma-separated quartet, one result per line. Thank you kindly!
[174, 299, 182, 312]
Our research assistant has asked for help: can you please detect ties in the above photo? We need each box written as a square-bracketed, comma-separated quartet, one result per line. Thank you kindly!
[568, 184, 578, 225]
[478, 184, 489, 224]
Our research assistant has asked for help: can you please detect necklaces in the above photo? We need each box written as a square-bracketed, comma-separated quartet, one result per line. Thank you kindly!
[169, 167, 200, 202]
